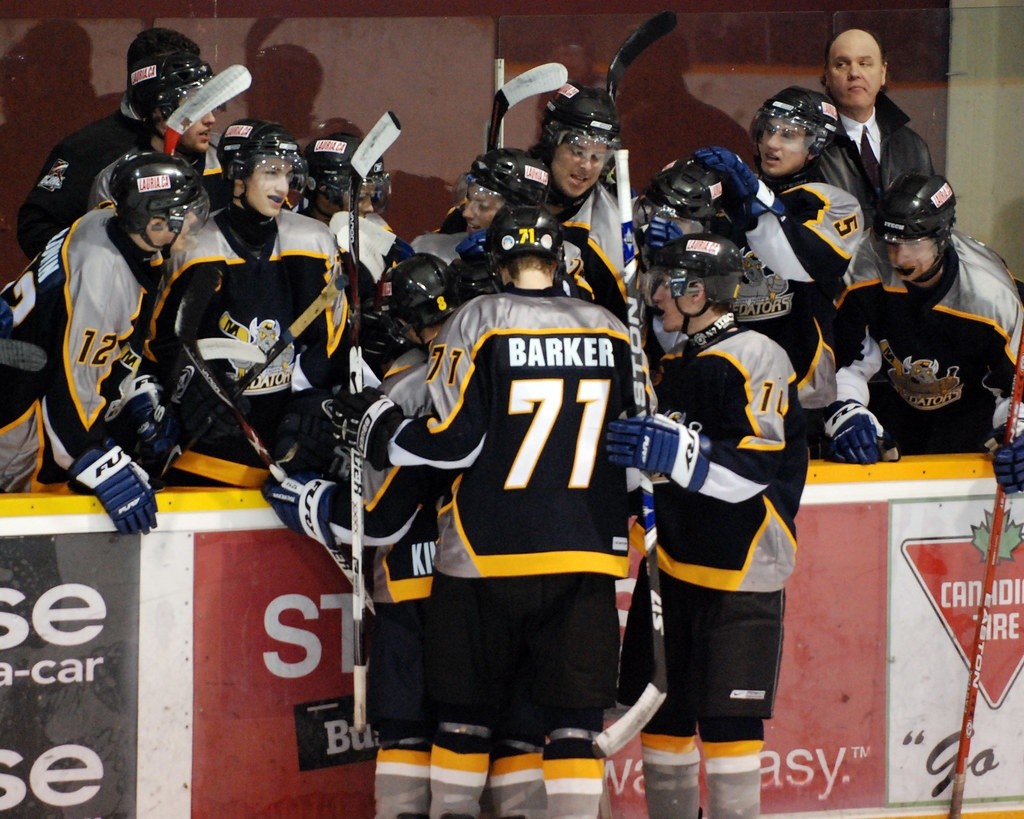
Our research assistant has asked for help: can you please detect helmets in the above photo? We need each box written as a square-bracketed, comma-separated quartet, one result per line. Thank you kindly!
[217, 117, 302, 180]
[645, 156, 737, 235]
[760, 86, 841, 155]
[386, 252, 459, 326]
[875, 174, 956, 254]
[656, 230, 745, 303]
[487, 203, 568, 261]
[109, 151, 205, 232]
[470, 147, 550, 205]
[542, 82, 619, 153]
[304, 133, 388, 199]
[126, 26, 228, 129]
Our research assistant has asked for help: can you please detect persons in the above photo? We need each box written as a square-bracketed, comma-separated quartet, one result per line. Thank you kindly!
[0, 28, 1024, 818]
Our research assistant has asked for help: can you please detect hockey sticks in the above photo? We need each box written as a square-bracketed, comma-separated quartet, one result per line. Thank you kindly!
[487, 57, 570, 150]
[162, 62, 254, 157]
[589, 6, 680, 763]
[148, 110, 403, 735]
[946, 315, 1024, 819]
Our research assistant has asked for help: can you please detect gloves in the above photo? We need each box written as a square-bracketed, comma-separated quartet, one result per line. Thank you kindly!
[66, 435, 160, 536]
[604, 414, 711, 493]
[331, 386, 406, 473]
[278, 390, 336, 454]
[161, 359, 250, 447]
[822, 399, 900, 466]
[124, 374, 187, 461]
[265, 469, 338, 554]
[995, 432, 1023, 494]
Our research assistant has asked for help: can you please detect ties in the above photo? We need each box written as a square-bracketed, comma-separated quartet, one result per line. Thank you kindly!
[859, 123, 882, 187]
